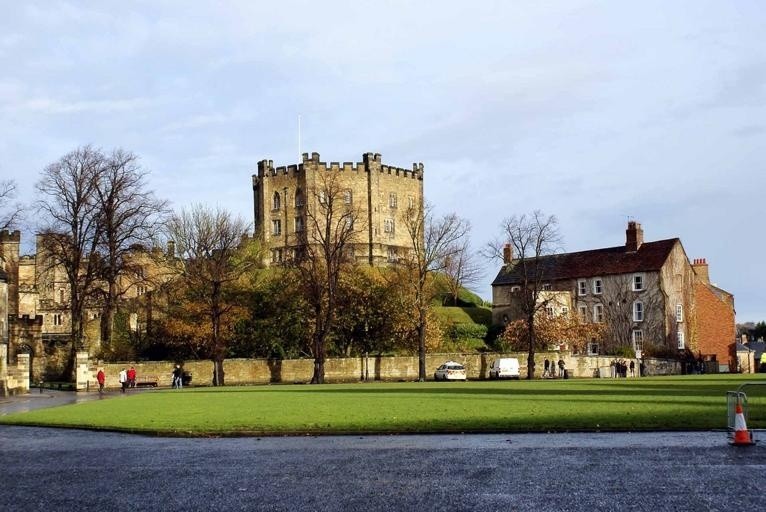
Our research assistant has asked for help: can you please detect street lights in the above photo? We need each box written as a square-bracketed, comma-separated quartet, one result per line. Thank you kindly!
[364, 321, 369, 381]
[642, 351, 645, 370]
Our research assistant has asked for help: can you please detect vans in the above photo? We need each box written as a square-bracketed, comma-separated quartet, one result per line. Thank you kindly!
[489, 358, 521, 380]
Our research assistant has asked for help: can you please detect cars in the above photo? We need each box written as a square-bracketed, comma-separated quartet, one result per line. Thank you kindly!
[434, 361, 466, 381]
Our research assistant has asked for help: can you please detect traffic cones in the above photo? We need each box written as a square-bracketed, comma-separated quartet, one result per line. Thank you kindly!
[728, 403, 757, 446]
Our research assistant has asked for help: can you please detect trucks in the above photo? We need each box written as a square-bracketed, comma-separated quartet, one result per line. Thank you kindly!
[647, 364, 671, 376]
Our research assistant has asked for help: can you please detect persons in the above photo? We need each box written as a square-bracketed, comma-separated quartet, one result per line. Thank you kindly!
[97, 368, 106, 393]
[610, 360, 648, 378]
[38, 375, 46, 393]
[558, 357, 565, 378]
[686, 360, 705, 374]
[170, 363, 183, 388]
[120, 367, 136, 393]
[542, 358, 552, 377]
